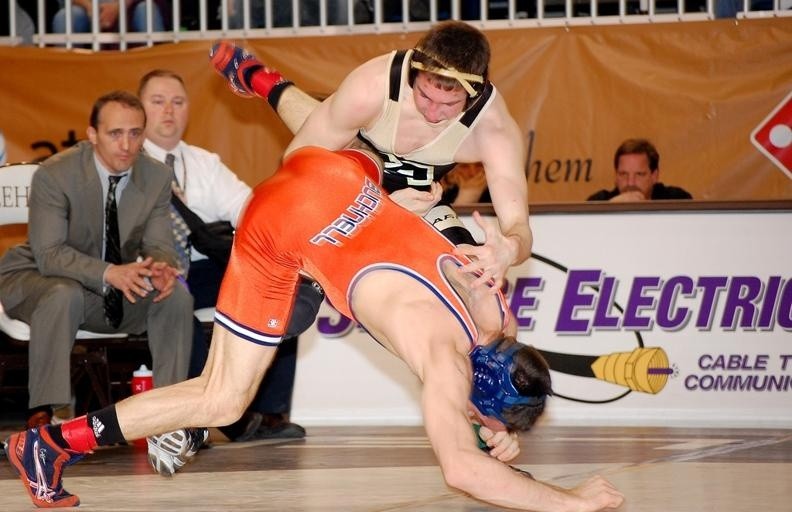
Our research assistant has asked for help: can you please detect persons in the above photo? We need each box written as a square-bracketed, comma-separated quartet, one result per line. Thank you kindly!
[53, 1, 167, 49]
[583, 137, 693, 201]
[133, 68, 311, 445]
[1, 40, 624, 511]
[439, 162, 492, 204]
[136, 16, 542, 480]
[0, 89, 209, 453]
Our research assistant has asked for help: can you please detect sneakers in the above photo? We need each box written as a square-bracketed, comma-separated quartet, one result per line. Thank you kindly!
[208, 39, 262, 100]
[4, 425, 81, 508]
[230, 410, 264, 442]
[146, 427, 209, 477]
[258, 414, 307, 441]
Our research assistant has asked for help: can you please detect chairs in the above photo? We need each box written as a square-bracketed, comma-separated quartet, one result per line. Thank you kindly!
[1, 154, 132, 435]
[193, 304, 217, 326]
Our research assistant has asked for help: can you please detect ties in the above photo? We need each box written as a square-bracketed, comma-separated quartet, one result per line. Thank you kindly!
[104, 174, 123, 328]
[165, 154, 191, 281]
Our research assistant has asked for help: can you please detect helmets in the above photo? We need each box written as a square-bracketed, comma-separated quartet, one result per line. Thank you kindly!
[409, 30, 490, 113]
[466, 336, 545, 428]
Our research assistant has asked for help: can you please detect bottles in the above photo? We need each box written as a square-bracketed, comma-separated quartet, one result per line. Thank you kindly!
[130, 363, 153, 448]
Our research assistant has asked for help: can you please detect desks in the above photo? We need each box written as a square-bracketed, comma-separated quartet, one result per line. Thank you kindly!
[267, 199, 791, 437]
